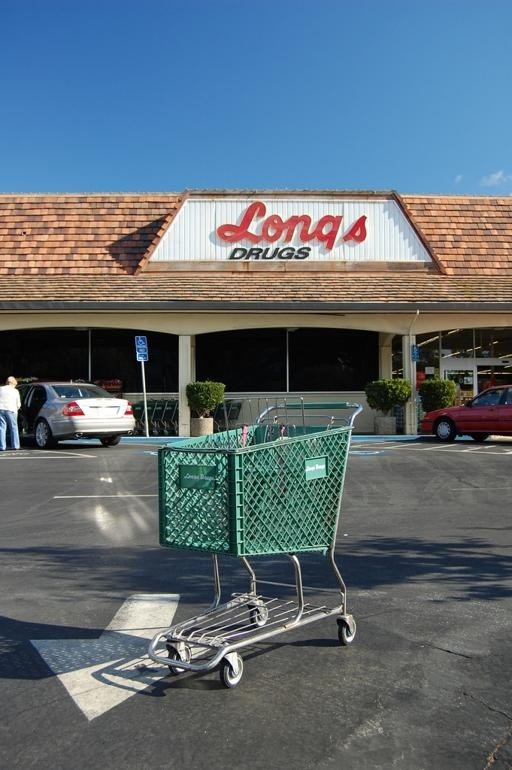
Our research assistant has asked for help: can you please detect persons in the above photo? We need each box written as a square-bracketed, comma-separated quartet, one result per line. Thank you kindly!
[0, 376, 23, 451]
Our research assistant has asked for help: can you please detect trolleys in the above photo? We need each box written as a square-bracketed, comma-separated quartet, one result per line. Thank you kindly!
[143, 401, 361, 684]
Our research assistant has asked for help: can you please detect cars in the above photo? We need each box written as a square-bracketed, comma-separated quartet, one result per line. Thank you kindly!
[417, 383, 511, 443]
[16, 377, 137, 448]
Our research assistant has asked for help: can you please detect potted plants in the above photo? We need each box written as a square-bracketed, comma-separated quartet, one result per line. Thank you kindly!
[185, 376, 229, 437]
[360, 374, 412, 435]
